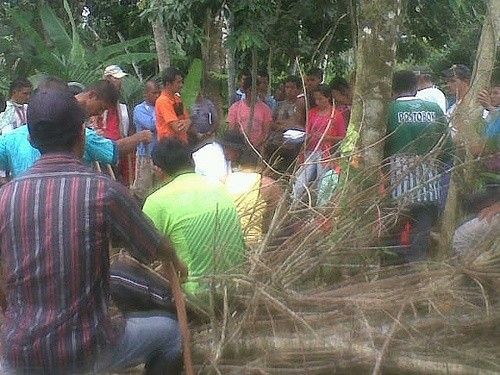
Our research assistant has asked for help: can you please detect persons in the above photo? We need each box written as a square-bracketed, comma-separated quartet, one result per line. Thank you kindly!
[0, 90, 189, 375]
[0, 65, 153, 189]
[110, 64, 500, 322]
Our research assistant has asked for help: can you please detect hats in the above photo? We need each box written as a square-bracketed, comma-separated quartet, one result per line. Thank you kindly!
[412, 66, 434, 75]
[26, 88, 89, 146]
[441, 64, 471, 79]
[104, 65, 129, 79]
